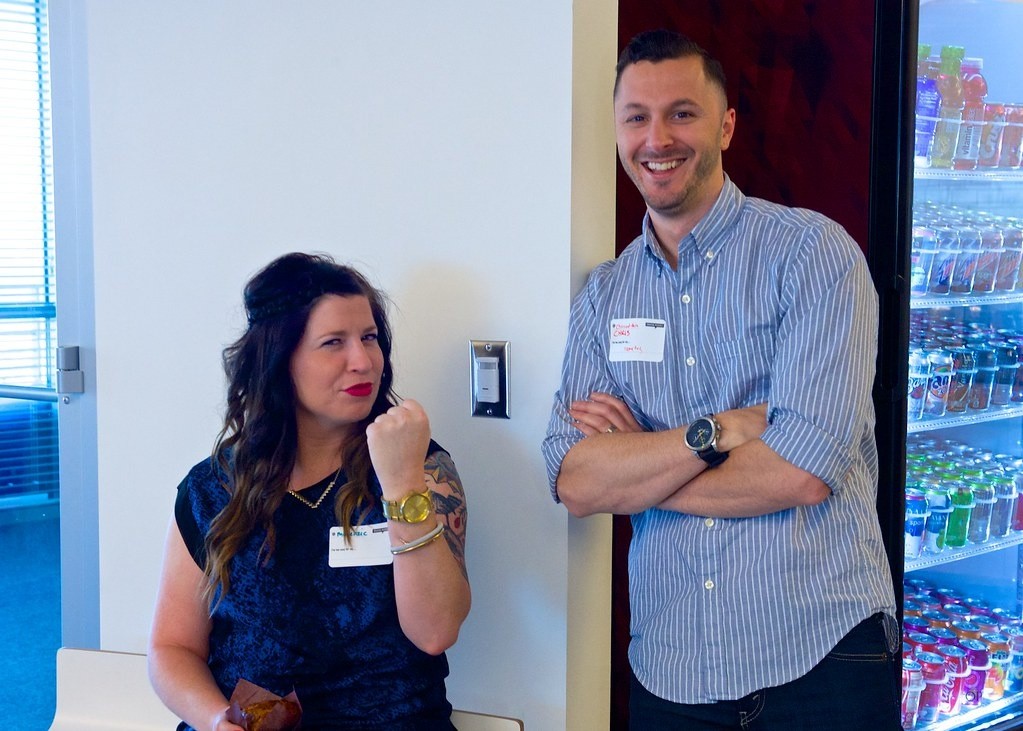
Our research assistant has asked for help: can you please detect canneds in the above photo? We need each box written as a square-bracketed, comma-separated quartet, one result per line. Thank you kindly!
[977, 101, 1023, 167]
[904, 205, 1023, 730]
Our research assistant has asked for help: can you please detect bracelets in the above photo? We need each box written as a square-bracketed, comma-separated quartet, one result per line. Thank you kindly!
[391, 520, 444, 554]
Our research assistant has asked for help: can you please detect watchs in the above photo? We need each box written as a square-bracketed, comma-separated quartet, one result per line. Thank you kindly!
[380, 485, 433, 526]
[683, 410, 729, 470]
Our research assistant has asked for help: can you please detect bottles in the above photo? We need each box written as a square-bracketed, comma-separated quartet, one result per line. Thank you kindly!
[953, 57, 988, 173]
[915, 56, 942, 172]
[930, 44, 967, 168]
[916, 44, 931, 94]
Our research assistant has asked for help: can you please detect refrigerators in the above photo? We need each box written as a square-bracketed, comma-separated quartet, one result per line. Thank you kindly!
[604, 0, 1022, 731]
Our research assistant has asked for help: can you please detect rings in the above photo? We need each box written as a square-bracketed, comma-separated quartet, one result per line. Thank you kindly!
[605, 424, 618, 434]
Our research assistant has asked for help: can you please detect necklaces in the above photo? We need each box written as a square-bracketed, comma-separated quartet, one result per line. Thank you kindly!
[285, 460, 343, 510]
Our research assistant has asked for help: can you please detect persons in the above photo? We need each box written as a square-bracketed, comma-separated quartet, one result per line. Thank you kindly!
[145, 252, 472, 730]
[542, 32, 907, 730]
